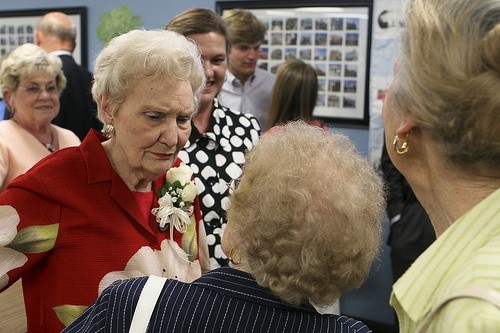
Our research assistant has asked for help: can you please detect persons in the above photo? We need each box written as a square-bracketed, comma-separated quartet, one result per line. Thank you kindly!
[383, 0, 500, 333]
[379, 129, 436, 333]
[62, 121, 386, 333]
[0, 12, 328, 194]
[164, 8, 260, 271]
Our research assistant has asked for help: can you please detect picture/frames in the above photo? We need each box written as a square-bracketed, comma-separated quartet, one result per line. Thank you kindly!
[216, 0, 372, 130]
[0, 6, 89, 100]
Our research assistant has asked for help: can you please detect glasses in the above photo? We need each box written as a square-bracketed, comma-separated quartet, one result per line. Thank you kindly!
[16, 82, 60, 94]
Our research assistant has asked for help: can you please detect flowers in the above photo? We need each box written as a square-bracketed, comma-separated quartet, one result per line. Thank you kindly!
[151, 163, 199, 244]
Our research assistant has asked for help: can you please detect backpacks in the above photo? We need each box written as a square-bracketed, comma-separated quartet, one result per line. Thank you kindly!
[54, 67, 103, 136]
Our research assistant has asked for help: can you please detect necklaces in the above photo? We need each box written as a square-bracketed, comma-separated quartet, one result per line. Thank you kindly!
[34, 131, 55, 153]
